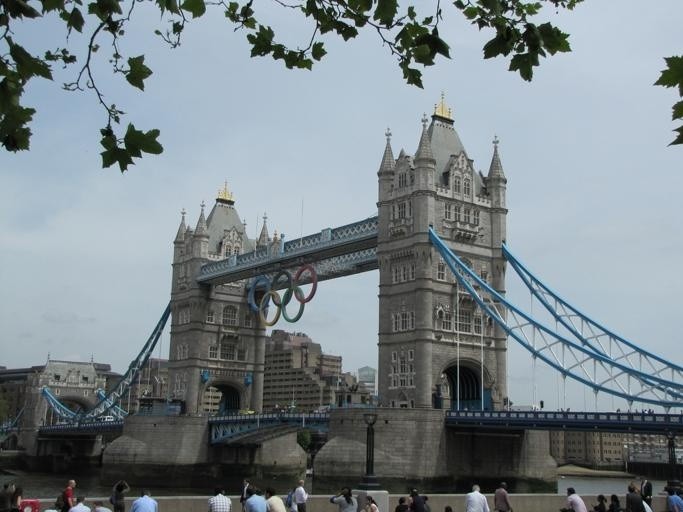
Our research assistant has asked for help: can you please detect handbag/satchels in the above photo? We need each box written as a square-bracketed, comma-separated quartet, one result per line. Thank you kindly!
[109, 493, 116, 506]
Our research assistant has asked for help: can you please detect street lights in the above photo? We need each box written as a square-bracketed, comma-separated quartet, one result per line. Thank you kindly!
[360, 413, 380, 490]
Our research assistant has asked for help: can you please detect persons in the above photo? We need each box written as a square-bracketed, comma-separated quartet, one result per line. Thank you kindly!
[644, 409, 647, 413]
[557, 475, 682, 512]
[648, 408, 654, 414]
[616, 408, 620, 420]
[0, 476, 160, 511]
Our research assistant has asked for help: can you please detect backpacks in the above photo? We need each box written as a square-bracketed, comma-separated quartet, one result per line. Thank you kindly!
[285, 495, 293, 508]
[55, 488, 68, 509]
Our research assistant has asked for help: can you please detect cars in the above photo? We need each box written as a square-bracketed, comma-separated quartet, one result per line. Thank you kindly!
[313, 405, 330, 414]
[236, 407, 258, 415]
[55, 415, 114, 425]
